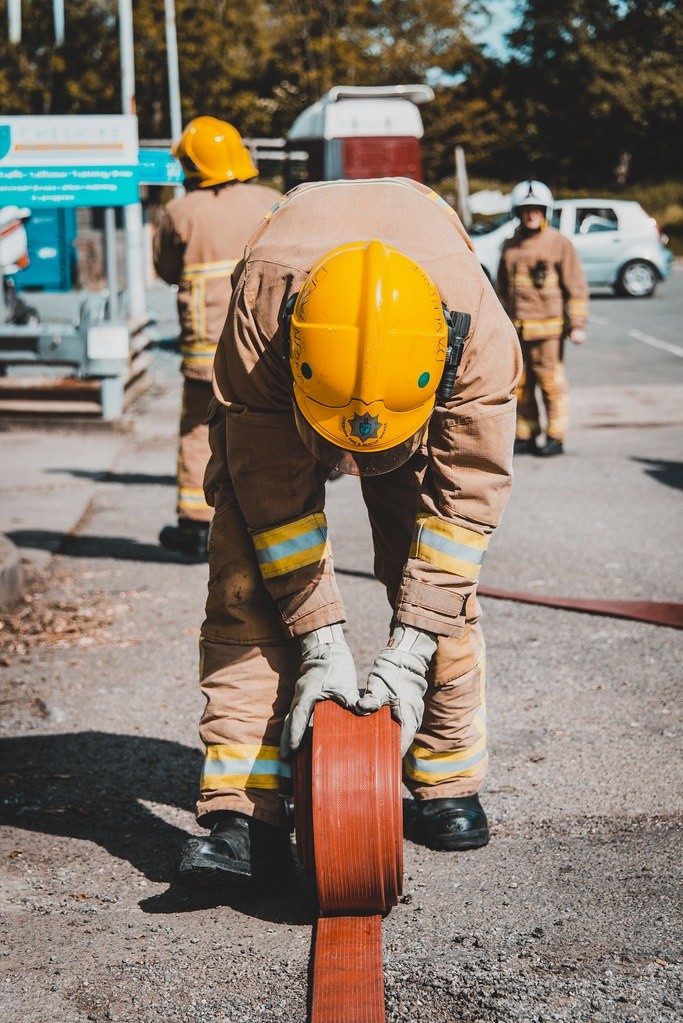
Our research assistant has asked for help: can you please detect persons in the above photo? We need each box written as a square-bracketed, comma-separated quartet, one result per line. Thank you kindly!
[178, 175, 525, 878]
[152, 116, 284, 566]
[496, 179, 590, 458]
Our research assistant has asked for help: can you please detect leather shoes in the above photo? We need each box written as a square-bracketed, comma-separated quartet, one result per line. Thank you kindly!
[159, 519, 210, 558]
[417, 793, 489, 850]
[178, 811, 297, 888]
[536, 438, 563, 456]
[514, 437, 535, 453]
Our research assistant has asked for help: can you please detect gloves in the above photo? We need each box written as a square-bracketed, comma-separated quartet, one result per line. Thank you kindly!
[357, 620, 440, 760]
[279, 623, 371, 761]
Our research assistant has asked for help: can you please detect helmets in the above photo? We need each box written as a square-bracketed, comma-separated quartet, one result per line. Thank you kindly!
[291, 240, 448, 452]
[172, 116, 260, 189]
[510, 180, 554, 220]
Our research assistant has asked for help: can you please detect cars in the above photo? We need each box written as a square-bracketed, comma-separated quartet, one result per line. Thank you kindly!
[468, 195, 674, 301]
